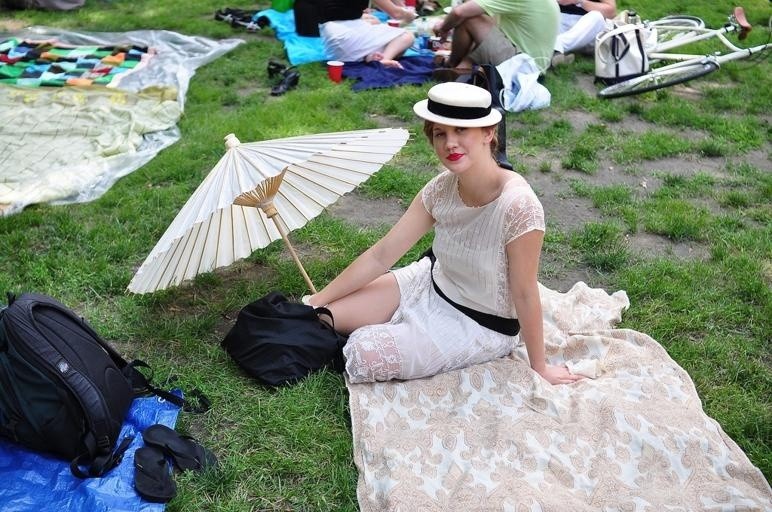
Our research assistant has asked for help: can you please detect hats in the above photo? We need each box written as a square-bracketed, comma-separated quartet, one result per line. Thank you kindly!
[413, 81, 503, 129]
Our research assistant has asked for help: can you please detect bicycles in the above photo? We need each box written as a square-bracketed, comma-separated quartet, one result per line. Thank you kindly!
[594, 3, 772, 100]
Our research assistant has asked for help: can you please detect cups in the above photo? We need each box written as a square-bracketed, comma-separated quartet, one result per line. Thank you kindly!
[326, 61, 346, 83]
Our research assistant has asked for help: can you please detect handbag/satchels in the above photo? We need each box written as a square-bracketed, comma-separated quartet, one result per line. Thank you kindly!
[220, 290, 348, 387]
[468, 53, 551, 112]
[594, 17, 650, 80]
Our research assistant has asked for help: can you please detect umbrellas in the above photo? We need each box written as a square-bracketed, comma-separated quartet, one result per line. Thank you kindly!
[124, 127, 415, 296]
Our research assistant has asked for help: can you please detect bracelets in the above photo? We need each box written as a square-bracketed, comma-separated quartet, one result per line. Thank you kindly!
[302, 294, 328, 310]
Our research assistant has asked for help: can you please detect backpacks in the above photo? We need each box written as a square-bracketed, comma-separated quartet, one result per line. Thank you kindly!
[0, 293, 155, 480]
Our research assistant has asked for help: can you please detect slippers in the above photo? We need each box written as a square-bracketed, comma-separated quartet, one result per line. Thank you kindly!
[130, 424, 221, 502]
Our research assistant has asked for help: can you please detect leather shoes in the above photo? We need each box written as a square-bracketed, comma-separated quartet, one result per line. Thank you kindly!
[267, 61, 301, 96]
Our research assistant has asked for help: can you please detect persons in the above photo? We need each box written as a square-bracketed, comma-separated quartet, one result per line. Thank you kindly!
[302, 81, 585, 386]
